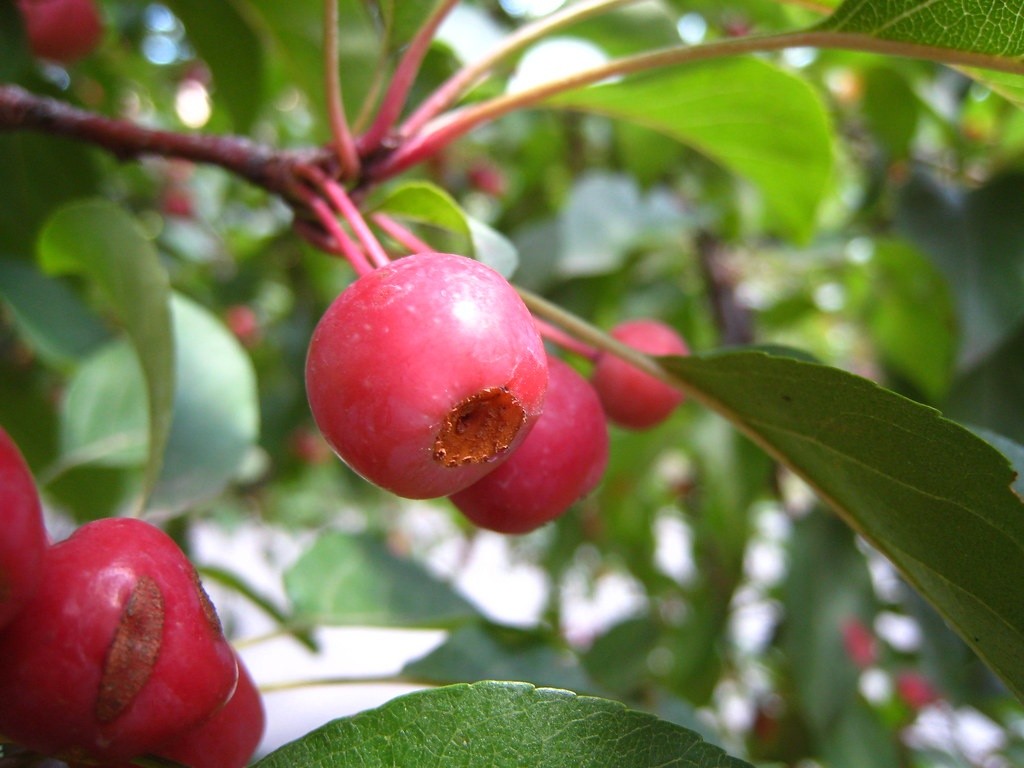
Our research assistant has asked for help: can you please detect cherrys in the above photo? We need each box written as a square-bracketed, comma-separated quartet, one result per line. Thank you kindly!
[0, 0, 690, 768]
